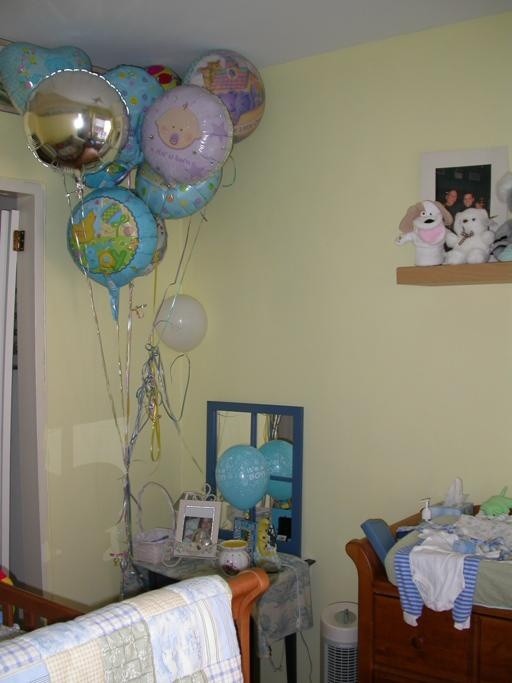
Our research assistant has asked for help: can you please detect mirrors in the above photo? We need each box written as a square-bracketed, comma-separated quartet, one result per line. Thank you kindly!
[204, 401, 303, 564]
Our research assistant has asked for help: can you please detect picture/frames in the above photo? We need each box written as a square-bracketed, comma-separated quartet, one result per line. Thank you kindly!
[173, 499, 223, 559]
[420, 144, 509, 252]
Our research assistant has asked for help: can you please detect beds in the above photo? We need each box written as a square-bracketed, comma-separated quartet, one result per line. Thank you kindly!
[0, 568, 271, 683]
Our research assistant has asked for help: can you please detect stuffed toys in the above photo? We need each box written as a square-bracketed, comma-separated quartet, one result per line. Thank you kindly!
[441, 208, 497, 263]
[394, 200, 456, 267]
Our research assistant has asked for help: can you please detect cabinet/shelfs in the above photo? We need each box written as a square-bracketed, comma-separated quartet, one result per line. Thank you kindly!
[345, 508, 512, 683]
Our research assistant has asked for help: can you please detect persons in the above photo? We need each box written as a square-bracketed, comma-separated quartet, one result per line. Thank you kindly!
[192, 517, 213, 544]
[460, 193, 483, 211]
[443, 189, 461, 215]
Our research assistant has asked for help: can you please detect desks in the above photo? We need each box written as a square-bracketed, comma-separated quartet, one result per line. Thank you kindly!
[138, 557, 317, 683]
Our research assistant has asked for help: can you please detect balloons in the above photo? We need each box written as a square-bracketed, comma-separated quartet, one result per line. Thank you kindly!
[155, 293, 208, 351]
[66, 186, 160, 290]
[100, 66, 165, 171]
[124, 64, 181, 93]
[215, 442, 270, 512]
[73, 164, 131, 189]
[0, 41, 93, 116]
[135, 150, 223, 219]
[139, 85, 234, 184]
[25, 69, 133, 177]
[260, 439, 294, 502]
[183, 48, 265, 142]
